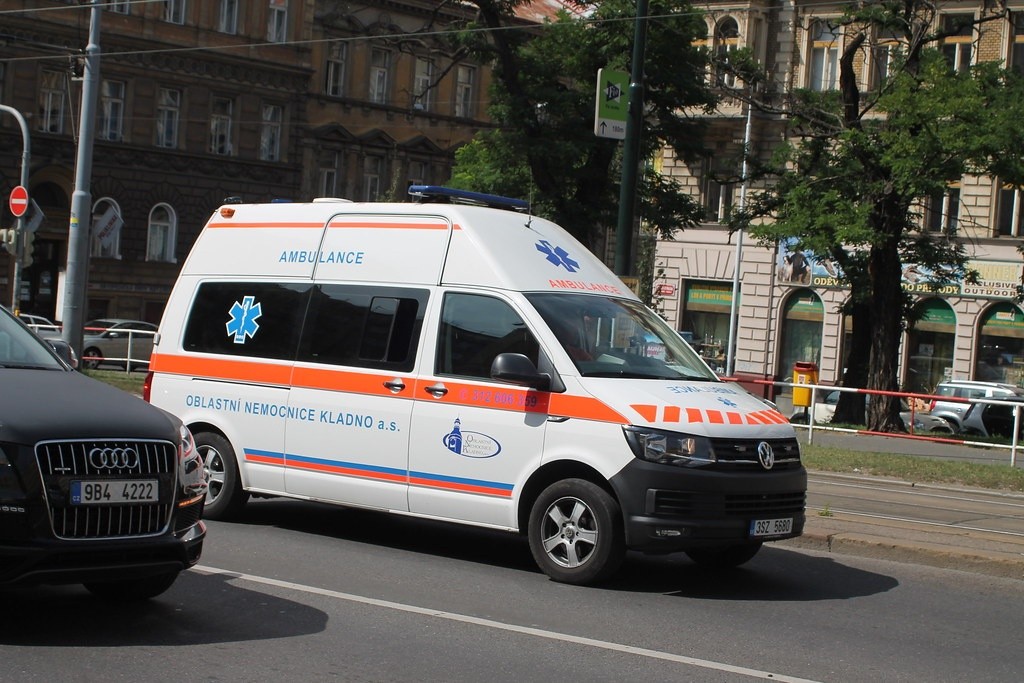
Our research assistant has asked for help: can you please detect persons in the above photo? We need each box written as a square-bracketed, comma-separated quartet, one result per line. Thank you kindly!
[548, 310, 592, 361]
[784, 250, 810, 282]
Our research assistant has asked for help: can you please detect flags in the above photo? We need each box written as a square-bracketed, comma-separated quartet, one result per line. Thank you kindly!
[10, 199, 44, 231]
[95, 207, 122, 250]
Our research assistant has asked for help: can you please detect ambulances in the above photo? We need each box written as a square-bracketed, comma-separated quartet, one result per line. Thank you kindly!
[149, 184, 808, 585]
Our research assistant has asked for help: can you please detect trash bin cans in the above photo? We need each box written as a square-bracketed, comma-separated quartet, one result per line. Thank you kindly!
[793, 360, 819, 407]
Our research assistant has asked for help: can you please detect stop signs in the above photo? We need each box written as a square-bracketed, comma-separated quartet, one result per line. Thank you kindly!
[9, 186, 28, 217]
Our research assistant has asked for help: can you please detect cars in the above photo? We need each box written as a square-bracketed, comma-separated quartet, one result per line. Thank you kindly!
[929, 379, 1024, 441]
[796, 391, 954, 439]
[962, 395, 1024, 439]
[17, 313, 60, 337]
[81, 319, 159, 370]
[0, 306, 211, 599]
